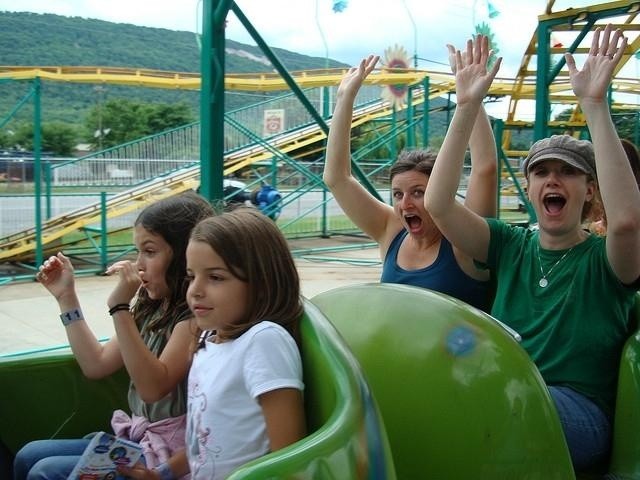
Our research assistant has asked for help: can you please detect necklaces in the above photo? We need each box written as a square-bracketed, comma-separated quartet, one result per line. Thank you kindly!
[534, 230, 578, 289]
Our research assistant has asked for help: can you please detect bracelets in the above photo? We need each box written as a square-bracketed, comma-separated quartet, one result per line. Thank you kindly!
[110, 303, 129, 311]
[110, 307, 130, 315]
[151, 463, 173, 480]
[60, 309, 85, 325]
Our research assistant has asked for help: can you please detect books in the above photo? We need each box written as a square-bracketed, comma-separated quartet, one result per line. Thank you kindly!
[66, 431, 144, 479]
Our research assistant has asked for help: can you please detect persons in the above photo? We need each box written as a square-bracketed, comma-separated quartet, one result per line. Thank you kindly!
[322, 44, 499, 313]
[422, 22, 640, 480]
[118, 207, 308, 480]
[11, 192, 222, 480]
[588, 137, 640, 236]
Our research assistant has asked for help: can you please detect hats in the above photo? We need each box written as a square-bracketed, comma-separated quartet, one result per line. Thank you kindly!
[521, 134, 596, 183]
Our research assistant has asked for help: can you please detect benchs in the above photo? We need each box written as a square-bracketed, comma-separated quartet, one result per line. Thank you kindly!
[0, 287, 418, 480]
[613, 329, 640, 477]
